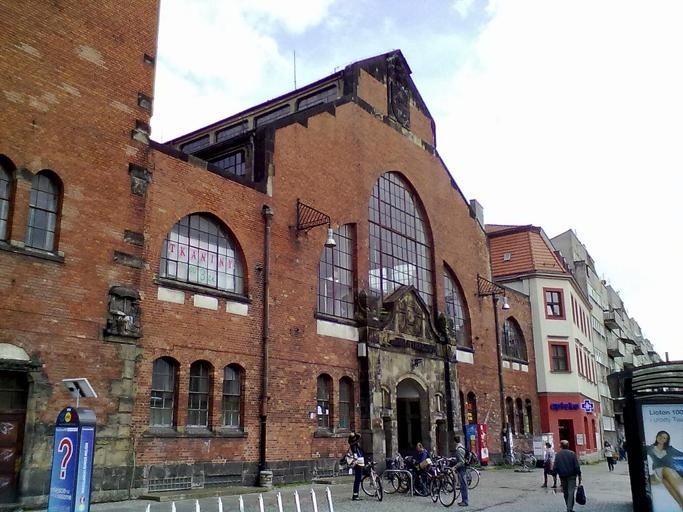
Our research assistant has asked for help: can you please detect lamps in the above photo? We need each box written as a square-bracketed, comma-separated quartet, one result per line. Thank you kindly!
[324, 223, 336, 248]
[502, 296, 509, 310]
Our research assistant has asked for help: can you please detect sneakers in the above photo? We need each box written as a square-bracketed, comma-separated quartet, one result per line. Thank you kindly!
[458, 501, 468, 506]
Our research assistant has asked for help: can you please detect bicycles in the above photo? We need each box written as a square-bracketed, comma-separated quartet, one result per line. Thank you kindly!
[359, 455, 383, 503]
[376, 451, 481, 507]
[502, 445, 537, 473]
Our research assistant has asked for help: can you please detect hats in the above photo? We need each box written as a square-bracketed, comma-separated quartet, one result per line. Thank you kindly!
[348, 432, 362, 444]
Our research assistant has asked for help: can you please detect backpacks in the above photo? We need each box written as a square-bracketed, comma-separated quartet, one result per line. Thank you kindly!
[458, 445, 478, 465]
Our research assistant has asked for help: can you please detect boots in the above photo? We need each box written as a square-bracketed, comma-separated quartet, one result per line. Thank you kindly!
[541, 479, 546, 487]
[551, 481, 557, 488]
[352, 495, 364, 500]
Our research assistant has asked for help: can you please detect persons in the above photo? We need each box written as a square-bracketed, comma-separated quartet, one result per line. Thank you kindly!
[541, 443, 557, 488]
[602, 437, 627, 471]
[345, 432, 370, 500]
[554, 440, 582, 512]
[452, 436, 468, 506]
[415, 442, 429, 494]
[646, 431, 683, 509]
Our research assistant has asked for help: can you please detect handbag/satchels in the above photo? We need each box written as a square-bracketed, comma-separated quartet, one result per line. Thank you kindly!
[415, 458, 432, 472]
[576, 484, 586, 505]
